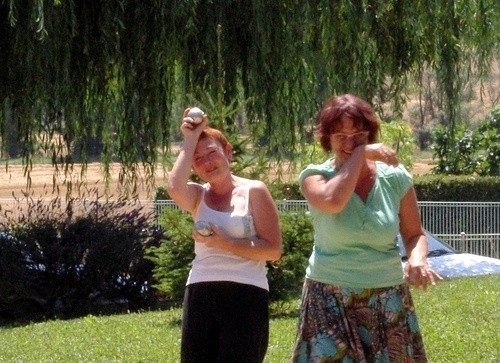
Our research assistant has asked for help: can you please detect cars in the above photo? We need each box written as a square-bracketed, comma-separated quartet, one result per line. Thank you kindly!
[396, 225, 499, 284]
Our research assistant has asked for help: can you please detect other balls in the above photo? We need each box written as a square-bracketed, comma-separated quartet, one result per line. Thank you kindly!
[188, 107, 203, 124]
[195, 221, 212, 236]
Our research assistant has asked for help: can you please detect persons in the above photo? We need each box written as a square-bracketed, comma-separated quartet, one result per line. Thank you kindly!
[290, 94, 444, 363]
[167, 108, 283, 363]
